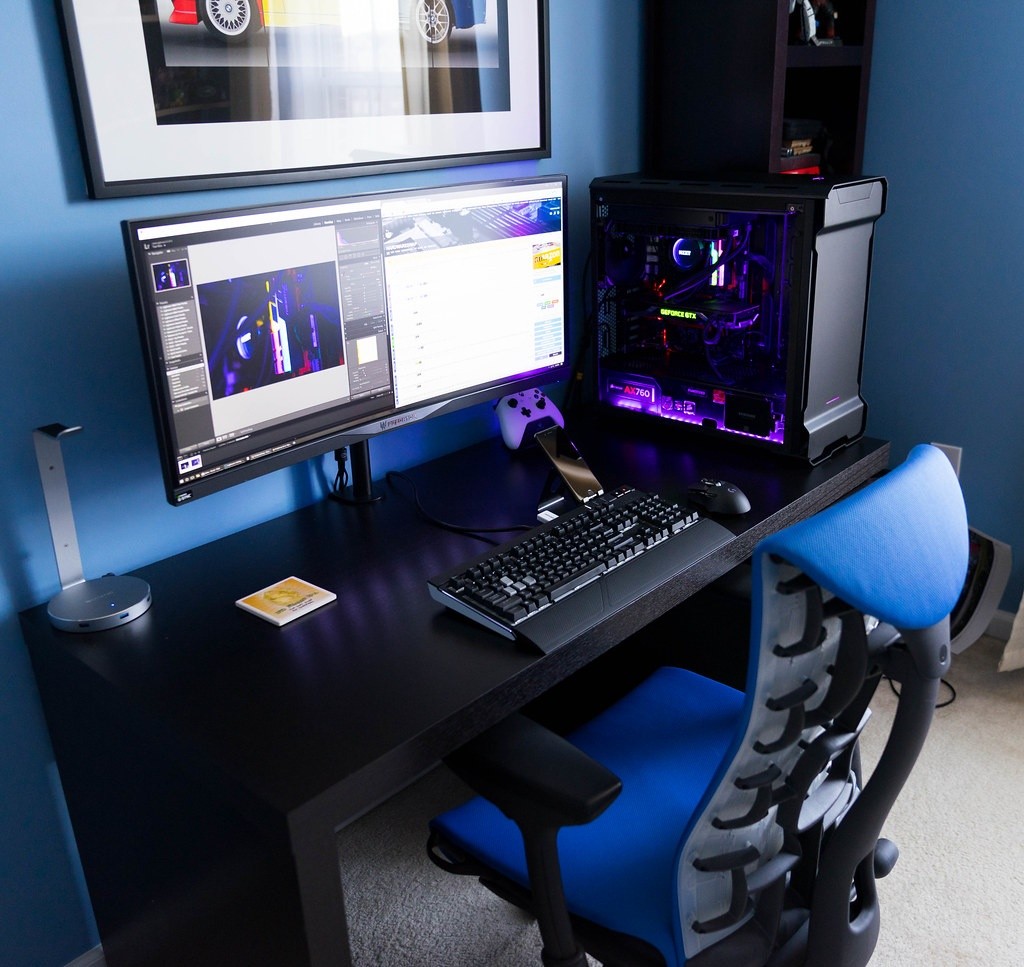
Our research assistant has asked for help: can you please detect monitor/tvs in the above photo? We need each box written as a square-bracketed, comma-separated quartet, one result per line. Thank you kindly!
[123, 170, 576, 507]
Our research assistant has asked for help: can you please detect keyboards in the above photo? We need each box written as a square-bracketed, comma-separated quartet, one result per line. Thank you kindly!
[427, 485, 736, 658]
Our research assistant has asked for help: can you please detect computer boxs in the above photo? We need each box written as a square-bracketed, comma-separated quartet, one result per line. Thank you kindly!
[590, 168, 889, 470]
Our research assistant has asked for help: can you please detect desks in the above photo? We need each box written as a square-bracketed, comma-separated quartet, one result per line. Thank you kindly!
[17, 402, 889, 967]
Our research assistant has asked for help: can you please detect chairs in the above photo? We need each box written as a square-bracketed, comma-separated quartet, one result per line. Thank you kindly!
[424, 444, 972, 967]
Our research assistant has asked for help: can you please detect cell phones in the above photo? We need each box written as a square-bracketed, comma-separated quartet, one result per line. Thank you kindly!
[532, 424, 604, 503]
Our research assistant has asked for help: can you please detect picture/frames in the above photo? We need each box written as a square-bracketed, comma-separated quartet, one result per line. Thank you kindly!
[54, 0, 552, 201]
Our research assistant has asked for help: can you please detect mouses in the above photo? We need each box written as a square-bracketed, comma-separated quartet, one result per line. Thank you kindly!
[686, 478, 751, 515]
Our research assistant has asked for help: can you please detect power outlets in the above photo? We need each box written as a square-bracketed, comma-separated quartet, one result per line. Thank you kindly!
[931, 442, 963, 478]
